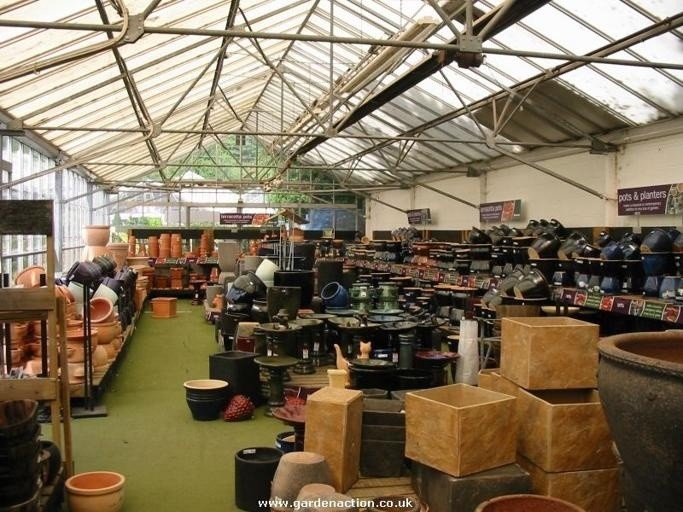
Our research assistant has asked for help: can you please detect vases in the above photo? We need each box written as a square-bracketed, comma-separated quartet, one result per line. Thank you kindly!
[594, 231, 616, 247]
[417, 314, 446, 349]
[369, 308, 404, 316]
[268, 287, 303, 322]
[417, 256, 427, 267]
[479, 307, 487, 317]
[219, 272, 236, 290]
[412, 242, 418, 254]
[92, 283, 118, 307]
[102, 344, 116, 362]
[469, 225, 489, 244]
[501, 246, 509, 252]
[11, 322, 26, 349]
[403, 288, 422, 301]
[584, 244, 601, 258]
[289, 243, 316, 271]
[338, 323, 382, 359]
[98, 256, 112, 271]
[416, 242, 432, 255]
[422, 281, 439, 290]
[514, 268, 551, 298]
[23, 359, 50, 375]
[468, 243, 490, 259]
[485, 225, 499, 236]
[71, 301, 76, 315]
[600, 276, 620, 295]
[414, 278, 423, 288]
[275, 431, 295, 455]
[373, 240, 388, 252]
[66, 320, 83, 332]
[240, 259, 249, 276]
[542, 306, 579, 315]
[455, 244, 470, 259]
[487, 308, 497, 336]
[445, 243, 453, 251]
[639, 252, 671, 277]
[83, 246, 108, 261]
[363, 399, 405, 412]
[600, 240, 623, 260]
[527, 231, 562, 258]
[396, 227, 407, 241]
[272, 405, 306, 451]
[552, 271, 569, 287]
[472, 304, 482, 317]
[479, 288, 497, 307]
[362, 412, 404, 426]
[500, 294, 516, 305]
[557, 240, 579, 259]
[386, 252, 402, 265]
[315, 248, 321, 259]
[376, 301, 399, 309]
[235, 447, 287, 511]
[131, 257, 156, 311]
[523, 445, 624, 512]
[398, 333, 416, 369]
[124, 308, 131, 325]
[619, 242, 640, 260]
[67, 362, 89, 384]
[255, 259, 280, 281]
[328, 318, 360, 364]
[82, 225, 111, 246]
[93, 346, 108, 372]
[221, 313, 250, 335]
[674, 233, 683, 253]
[170, 277, 183, 289]
[294, 483, 335, 512]
[402, 253, 414, 265]
[125, 290, 130, 305]
[210, 278, 218, 283]
[513, 264, 523, 273]
[512, 237, 533, 247]
[436, 305, 454, 318]
[29, 340, 42, 357]
[500, 317, 599, 390]
[365, 250, 376, 260]
[332, 240, 344, 249]
[223, 335, 234, 352]
[391, 228, 401, 241]
[318, 260, 343, 298]
[359, 425, 407, 477]
[450, 308, 465, 325]
[82, 322, 116, 344]
[209, 351, 260, 410]
[348, 248, 355, 261]
[147, 237, 158, 258]
[348, 360, 397, 390]
[533, 219, 549, 237]
[447, 335, 459, 380]
[114, 297, 121, 315]
[152, 297, 177, 317]
[321, 282, 350, 306]
[306, 313, 337, 366]
[359, 275, 371, 283]
[105, 256, 117, 268]
[499, 225, 510, 236]
[390, 277, 411, 295]
[111, 336, 122, 352]
[107, 279, 126, 299]
[566, 230, 593, 247]
[290, 318, 323, 375]
[368, 316, 403, 348]
[380, 321, 418, 352]
[474, 495, 585, 512]
[508, 228, 523, 237]
[259, 240, 279, 256]
[598, 260, 624, 276]
[349, 283, 374, 302]
[414, 350, 459, 387]
[115, 272, 133, 289]
[103, 256, 115, 271]
[170, 267, 184, 278]
[199, 274, 208, 281]
[439, 243, 446, 250]
[501, 297, 550, 317]
[225, 271, 267, 313]
[212, 251, 219, 257]
[236, 335, 256, 353]
[303, 388, 362, 494]
[57, 331, 94, 363]
[642, 275, 666, 298]
[155, 275, 168, 288]
[659, 276, 680, 298]
[360, 389, 388, 400]
[666, 229, 680, 242]
[121, 294, 126, 312]
[433, 285, 460, 306]
[66, 261, 93, 286]
[588, 258, 602, 274]
[411, 255, 421, 266]
[492, 265, 502, 279]
[390, 390, 406, 401]
[349, 302, 375, 314]
[439, 251, 454, 268]
[107, 244, 128, 269]
[373, 251, 387, 262]
[454, 288, 479, 310]
[120, 312, 127, 330]
[210, 267, 219, 277]
[502, 264, 513, 278]
[72, 329, 99, 352]
[244, 255, 269, 274]
[673, 253, 683, 276]
[198, 233, 209, 257]
[75, 304, 83, 314]
[251, 298, 270, 323]
[325, 248, 336, 258]
[423, 289, 435, 297]
[556, 259, 573, 272]
[371, 274, 391, 288]
[640, 228, 671, 253]
[128, 235, 136, 256]
[492, 246, 503, 254]
[183, 380, 229, 423]
[394, 369, 429, 389]
[625, 260, 643, 294]
[514, 296, 547, 306]
[82, 298, 115, 323]
[319, 242, 330, 257]
[205, 286, 223, 308]
[92, 257, 108, 273]
[312, 492, 357, 512]
[115, 321, 120, 338]
[453, 260, 472, 275]
[334, 249, 343, 258]
[416, 297, 431, 310]
[366, 496, 421, 512]
[254, 356, 299, 407]
[576, 274, 589, 289]
[404, 385, 517, 478]
[512, 246, 519, 254]
[189, 280, 206, 306]
[490, 230, 506, 246]
[274, 270, 316, 308]
[103, 277, 112, 286]
[377, 282, 401, 300]
[529, 259, 559, 284]
[429, 249, 441, 265]
[586, 276, 601, 293]
[270, 452, 328, 511]
[259, 323, 302, 382]
[500, 268, 527, 296]
[386, 241, 402, 252]
[617, 231, 641, 246]
[501, 236, 513, 246]
[320, 238, 332, 247]
[434, 243, 439, 248]
[355, 244, 370, 250]
[189, 273, 200, 282]
[368, 240, 375, 250]
[55, 278, 75, 302]
[14, 267, 46, 289]
[523, 264, 532, 277]
[313, 240, 321, 249]
[543, 219, 569, 237]
[516, 389, 614, 471]
[68, 281, 93, 305]
[372, 350, 392, 362]
[489, 293, 504, 309]
[66, 303, 73, 317]
[159, 234, 170, 258]
[469, 260, 490, 275]
[354, 249, 366, 261]
[596, 330, 683, 508]
[171, 234, 181, 258]
[523, 220, 540, 236]
[577, 257, 588, 274]
[328, 369, 348, 387]
[65, 472, 125, 512]
[33, 321, 49, 335]
[411, 463, 530, 511]
[10, 348, 22, 368]
[400, 226, 419, 240]
